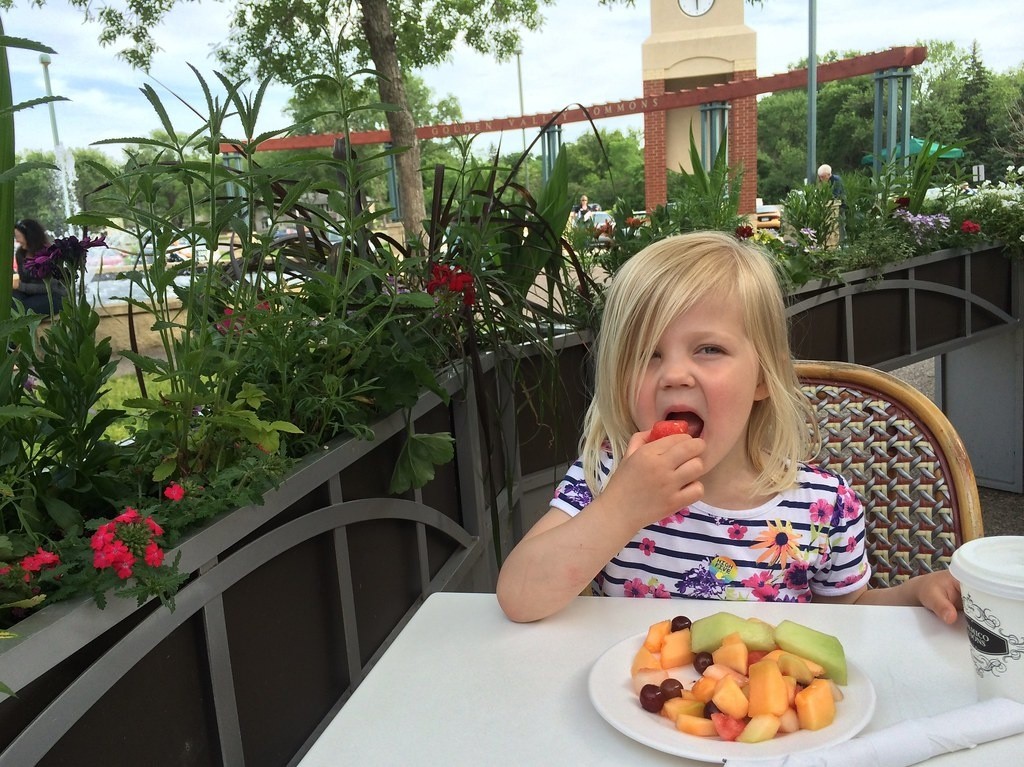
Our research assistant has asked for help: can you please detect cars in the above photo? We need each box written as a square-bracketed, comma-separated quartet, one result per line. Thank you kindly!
[169, 228, 221, 264]
[324, 227, 375, 257]
[88, 247, 125, 266]
[572, 211, 617, 231]
[757, 204, 784, 230]
[129, 242, 188, 265]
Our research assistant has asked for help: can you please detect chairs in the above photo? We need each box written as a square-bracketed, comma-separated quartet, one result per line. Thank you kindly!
[579, 360, 985, 596]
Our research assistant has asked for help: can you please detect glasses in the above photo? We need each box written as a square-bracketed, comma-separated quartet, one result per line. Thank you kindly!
[18, 221, 26, 228]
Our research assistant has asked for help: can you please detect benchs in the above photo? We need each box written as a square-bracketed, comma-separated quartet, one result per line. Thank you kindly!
[33, 288, 303, 359]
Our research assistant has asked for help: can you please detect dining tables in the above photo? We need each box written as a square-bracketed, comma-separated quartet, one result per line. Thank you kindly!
[298, 593, 1024, 767]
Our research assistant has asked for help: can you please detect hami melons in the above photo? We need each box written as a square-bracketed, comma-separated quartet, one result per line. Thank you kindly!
[632, 610, 848, 743]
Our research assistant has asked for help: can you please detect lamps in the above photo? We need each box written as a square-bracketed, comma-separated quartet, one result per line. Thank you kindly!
[972, 160, 985, 185]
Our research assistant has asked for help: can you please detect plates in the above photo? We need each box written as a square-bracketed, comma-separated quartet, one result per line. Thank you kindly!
[588, 633, 876, 764]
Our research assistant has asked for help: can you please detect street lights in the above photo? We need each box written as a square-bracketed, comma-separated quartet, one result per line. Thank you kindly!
[513, 49, 531, 192]
[39, 53, 58, 146]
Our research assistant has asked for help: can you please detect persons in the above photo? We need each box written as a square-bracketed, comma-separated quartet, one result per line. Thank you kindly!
[5, 218, 68, 355]
[814, 160, 849, 202]
[524, 195, 601, 224]
[954, 181, 975, 195]
[140, 233, 193, 265]
[496, 231, 979, 626]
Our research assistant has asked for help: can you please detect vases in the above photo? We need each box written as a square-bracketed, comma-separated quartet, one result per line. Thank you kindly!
[0, 234, 1024, 767]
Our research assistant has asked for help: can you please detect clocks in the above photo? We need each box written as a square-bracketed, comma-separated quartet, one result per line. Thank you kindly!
[678, 0, 715, 17]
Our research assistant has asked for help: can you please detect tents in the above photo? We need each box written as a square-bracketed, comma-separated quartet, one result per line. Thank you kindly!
[861, 136, 964, 191]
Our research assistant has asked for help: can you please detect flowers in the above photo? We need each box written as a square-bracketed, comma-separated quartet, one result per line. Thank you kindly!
[1, 23, 1024, 698]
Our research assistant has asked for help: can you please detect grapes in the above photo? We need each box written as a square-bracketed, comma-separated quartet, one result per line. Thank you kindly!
[693, 651, 714, 674]
[704, 699, 725, 718]
[641, 678, 683, 711]
[672, 616, 692, 634]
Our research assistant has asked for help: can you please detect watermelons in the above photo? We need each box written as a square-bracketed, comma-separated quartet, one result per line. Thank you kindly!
[745, 644, 782, 676]
[709, 711, 745, 741]
[649, 420, 689, 443]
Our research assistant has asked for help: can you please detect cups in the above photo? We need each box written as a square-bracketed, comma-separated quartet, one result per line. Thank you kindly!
[949, 535, 1024, 703]
